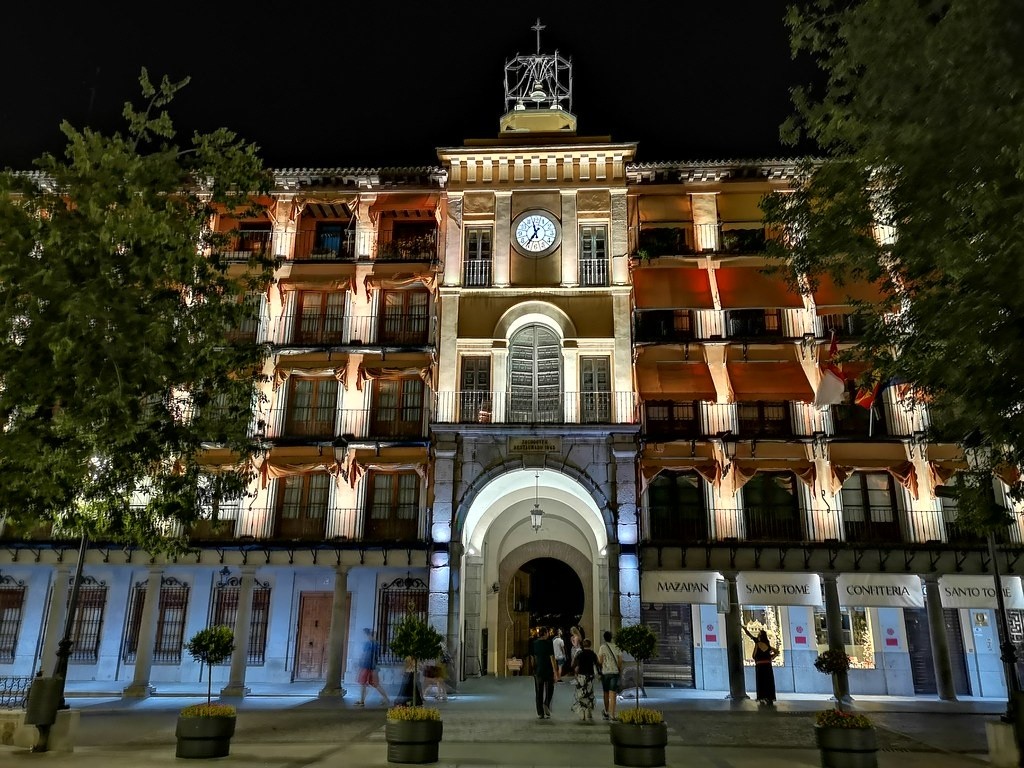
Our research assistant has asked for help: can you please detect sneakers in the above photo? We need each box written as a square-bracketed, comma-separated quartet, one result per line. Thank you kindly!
[557, 679, 565, 683]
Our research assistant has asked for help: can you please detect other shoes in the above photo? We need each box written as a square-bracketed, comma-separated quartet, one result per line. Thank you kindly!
[353, 701, 364, 705]
[538, 714, 544, 718]
[611, 718, 619, 723]
[603, 710, 609, 720]
[543, 703, 550, 716]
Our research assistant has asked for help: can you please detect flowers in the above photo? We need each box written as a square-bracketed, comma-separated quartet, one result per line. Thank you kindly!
[385, 705, 441, 721]
[816, 708, 874, 729]
[617, 707, 665, 725]
[183, 703, 236, 720]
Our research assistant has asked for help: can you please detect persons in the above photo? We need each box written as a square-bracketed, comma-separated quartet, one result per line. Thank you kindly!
[743, 627, 778, 706]
[529, 626, 622, 722]
[353, 628, 390, 707]
[394, 637, 453, 707]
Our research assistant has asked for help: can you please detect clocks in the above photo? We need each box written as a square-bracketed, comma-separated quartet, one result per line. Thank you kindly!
[516, 215, 556, 252]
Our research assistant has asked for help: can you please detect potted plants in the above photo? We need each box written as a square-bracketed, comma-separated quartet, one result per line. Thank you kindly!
[385, 598, 444, 764]
[610, 624, 667, 768]
[175, 625, 237, 759]
[812, 650, 879, 768]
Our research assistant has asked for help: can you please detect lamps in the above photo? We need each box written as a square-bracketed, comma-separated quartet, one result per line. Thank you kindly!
[335, 434, 348, 463]
[530, 475, 543, 533]
[721, 430, 738, 479]
